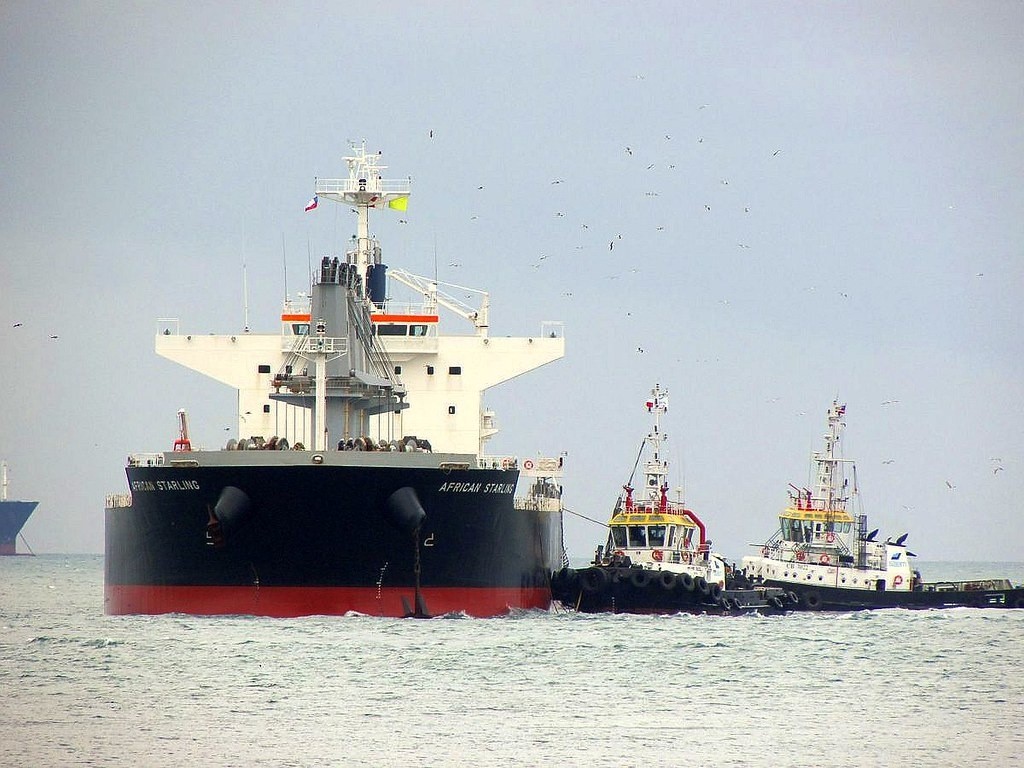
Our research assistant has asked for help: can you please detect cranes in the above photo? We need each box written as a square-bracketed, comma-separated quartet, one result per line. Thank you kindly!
[171, 406, 191, 452]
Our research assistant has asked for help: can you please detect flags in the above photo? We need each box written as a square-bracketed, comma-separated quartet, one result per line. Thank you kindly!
[304, 196, 318, 213]
[388, 195, 409, 212]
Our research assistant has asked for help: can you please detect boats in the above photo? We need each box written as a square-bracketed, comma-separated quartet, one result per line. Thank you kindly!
[738, 389, 1024, 615]
[557, 380, 800, 618]
[100, 138, 572, 622]
[0, 457, 42, 557]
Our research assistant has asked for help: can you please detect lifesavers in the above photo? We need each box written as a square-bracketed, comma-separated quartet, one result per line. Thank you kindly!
[682, 553, 688, 562]
[797, 551, 805, 561]
[652, 550, 663, 560]
[719, 580, 724, 590]
[894, 575, 902, 584]
[820, 554, 829, 565]
[826, 534, 834, 543]
